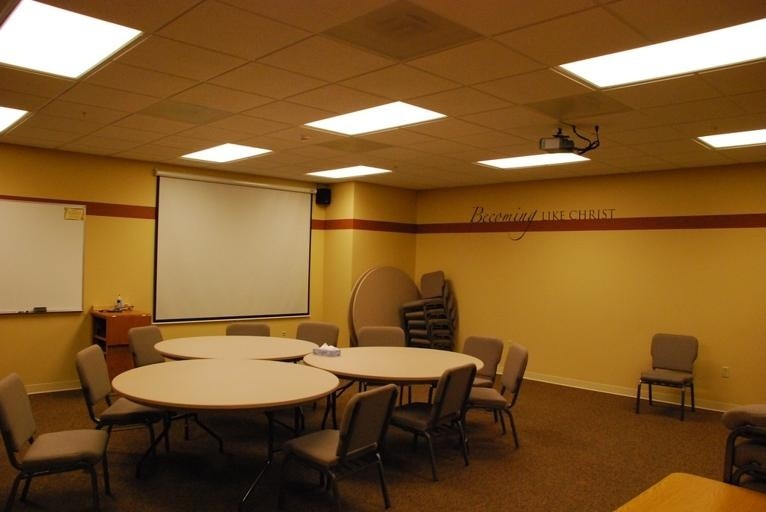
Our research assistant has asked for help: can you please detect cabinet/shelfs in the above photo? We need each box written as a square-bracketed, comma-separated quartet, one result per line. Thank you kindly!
[88, 307, 152, 398]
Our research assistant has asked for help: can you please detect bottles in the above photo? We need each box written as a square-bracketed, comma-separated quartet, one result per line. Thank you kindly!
[117, 295, 122, 308]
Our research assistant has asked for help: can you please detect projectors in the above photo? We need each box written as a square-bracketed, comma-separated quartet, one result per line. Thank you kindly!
[538, 136, 576, 155]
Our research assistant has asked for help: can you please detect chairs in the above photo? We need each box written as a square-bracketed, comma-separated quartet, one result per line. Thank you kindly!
[400, 270, 458, 351]
[635, 332, 699, 422]
[719, 403, 766, 491]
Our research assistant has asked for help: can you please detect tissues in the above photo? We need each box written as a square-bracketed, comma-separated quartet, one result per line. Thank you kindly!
[313, 343, 340, 357]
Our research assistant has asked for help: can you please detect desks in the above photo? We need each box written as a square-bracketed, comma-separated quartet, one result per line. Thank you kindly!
[611, 471, 766, 512]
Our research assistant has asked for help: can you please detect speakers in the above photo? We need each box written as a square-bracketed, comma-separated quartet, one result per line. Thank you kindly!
[316, 188, 331, 204]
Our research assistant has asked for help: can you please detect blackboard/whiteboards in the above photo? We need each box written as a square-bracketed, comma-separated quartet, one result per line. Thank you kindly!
[0, 198, 86, 314]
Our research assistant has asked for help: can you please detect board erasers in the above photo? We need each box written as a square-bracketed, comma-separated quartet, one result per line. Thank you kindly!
[34, 308, 47, 312]
